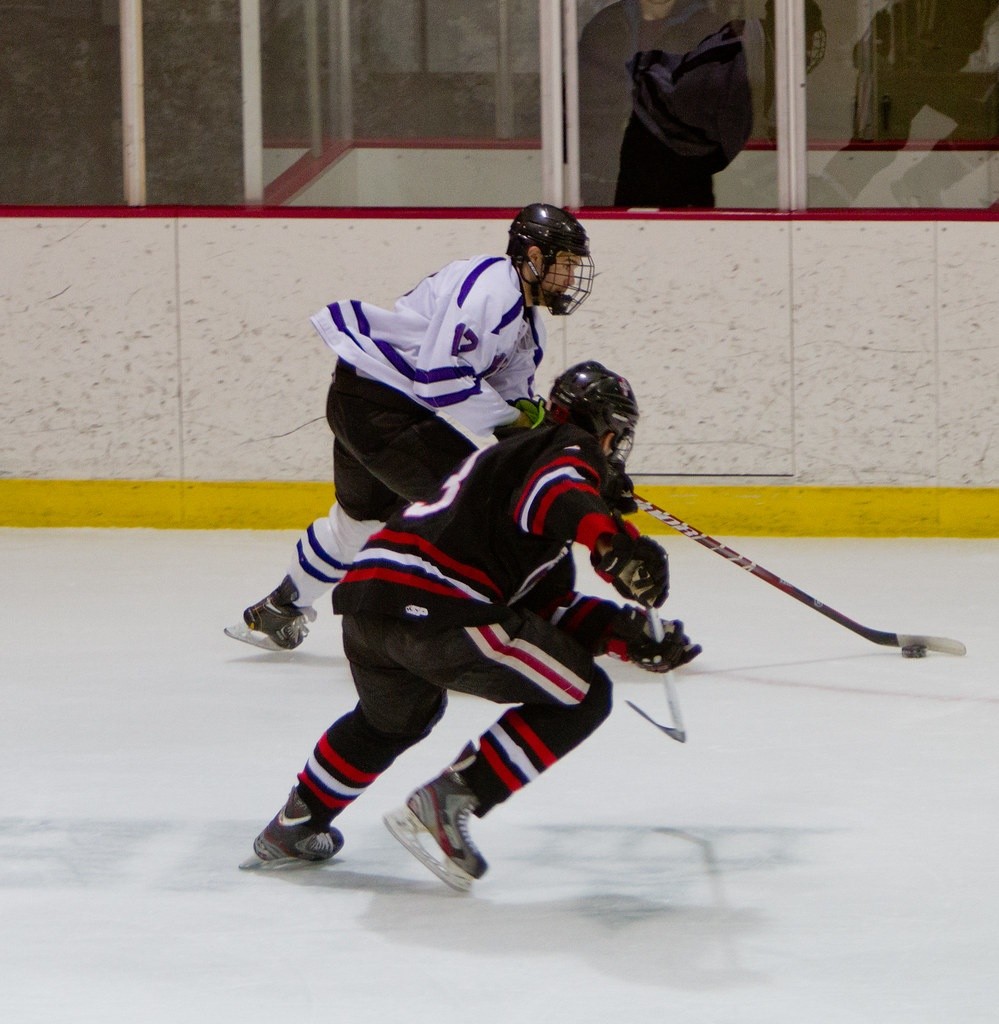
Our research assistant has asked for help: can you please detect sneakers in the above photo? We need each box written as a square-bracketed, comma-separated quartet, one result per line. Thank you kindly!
[238, 785, 344, 868]
[383, 740, 488, 892]
[224, 574, 316, 651]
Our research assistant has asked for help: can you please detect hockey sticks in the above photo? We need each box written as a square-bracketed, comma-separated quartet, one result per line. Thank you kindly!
[631, 493, 968, 657]
[625, 600, 688, 746]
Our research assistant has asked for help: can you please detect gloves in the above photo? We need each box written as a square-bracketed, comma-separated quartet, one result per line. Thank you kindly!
[493, 396, 550, 441]
[590, 530, 670, 608]
[604, 604, 702, 674]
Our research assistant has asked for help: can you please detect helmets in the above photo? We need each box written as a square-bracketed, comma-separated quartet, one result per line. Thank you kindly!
[549, 361, 639, 466]
[506, 202, 595, 317]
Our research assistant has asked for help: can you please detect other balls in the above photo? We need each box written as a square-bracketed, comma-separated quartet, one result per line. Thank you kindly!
[902, 644, 927, 657]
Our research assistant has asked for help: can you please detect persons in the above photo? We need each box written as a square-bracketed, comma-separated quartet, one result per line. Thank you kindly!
[222, 201, 598, 656]
[615, 0, 828, 210]
[559, 0, 756, 212]
[242, 359, 706, 898]
[802, 0, 999, 212]
